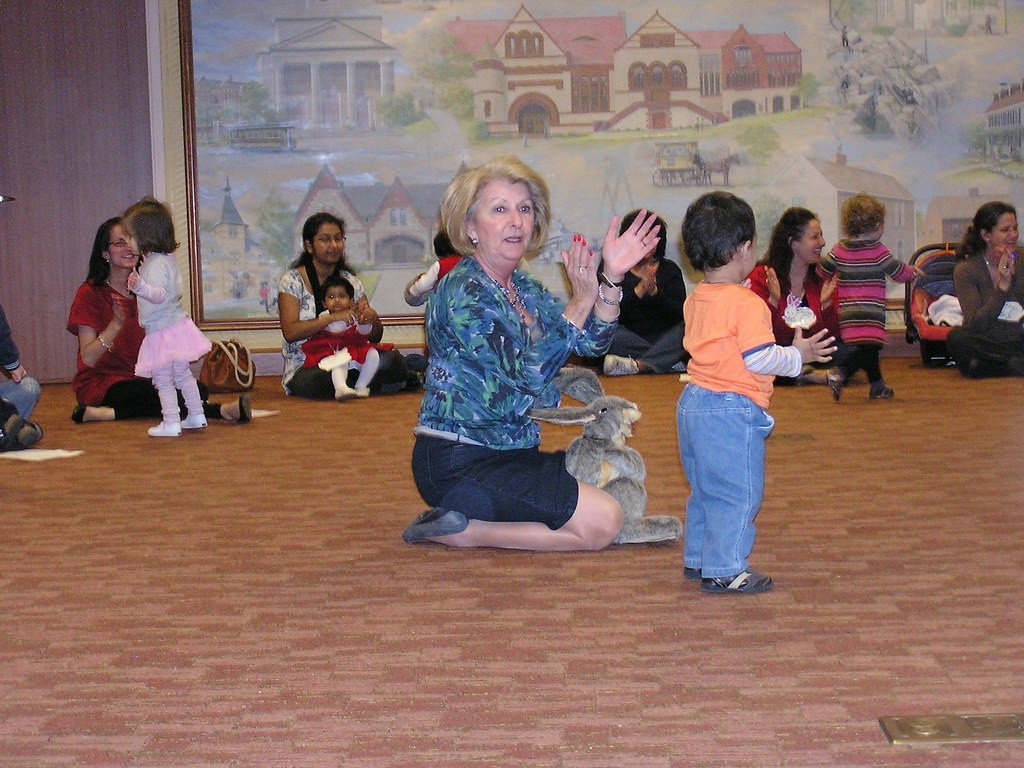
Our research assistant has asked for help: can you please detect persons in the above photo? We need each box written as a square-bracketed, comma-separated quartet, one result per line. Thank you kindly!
[945, 201, 1024, 380]
[596, 209, 689, 376]
[402, 153, 661, 550]
[66, 215, 252, 424]
[302, 276, 380, 400]
[278, 212, 421, 400]
[409, 228, 462, 296]
[121, 196, 212, 437]
[677, 192, 838, 594]
[747, 206, 841, 386]
[0, 300, 43, 450]
[815, 189, 927, 400]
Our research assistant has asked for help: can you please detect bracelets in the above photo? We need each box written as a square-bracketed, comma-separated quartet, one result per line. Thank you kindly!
[561, 313, 581, 331]
[598, 285, 623, 305]
[597, 271, 625, 292]
[596, 304, 621, 318]
[98, 331, 114, 353]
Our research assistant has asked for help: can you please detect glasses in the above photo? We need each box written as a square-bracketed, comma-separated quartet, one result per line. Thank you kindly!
[309, 235, 346, 244]
[104, 238, 127, 248]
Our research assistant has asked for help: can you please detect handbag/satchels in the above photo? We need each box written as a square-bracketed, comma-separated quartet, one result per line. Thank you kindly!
[197, 339, 256, 395]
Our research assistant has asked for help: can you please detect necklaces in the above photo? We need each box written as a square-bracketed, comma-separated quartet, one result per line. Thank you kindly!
[487, 275, 525, 309]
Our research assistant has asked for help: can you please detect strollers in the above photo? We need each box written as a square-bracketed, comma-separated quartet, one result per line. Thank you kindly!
[903, 242, 969, 369]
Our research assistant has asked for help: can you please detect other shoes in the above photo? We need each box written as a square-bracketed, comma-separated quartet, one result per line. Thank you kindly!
[334, 386, 356, 401]
[148, 421, 182, 437]
[795, 364, 814, 381]
[403, 505, 469, 544]
[235, 394, 253, 422]
[0, 414, 43, 450]
[404, 370, 422, 392]
[963, 356, 985, 379]
[71, 403, 87, 424]
[180, 414, 208, 429]
[870, 385, 894, 400]
[826, 367, 842, 402]
[356, 385, 370, 398]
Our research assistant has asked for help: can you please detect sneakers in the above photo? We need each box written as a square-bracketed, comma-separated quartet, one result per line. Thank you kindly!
[604, 354, 639, 378]
[684, 564, 772, 594]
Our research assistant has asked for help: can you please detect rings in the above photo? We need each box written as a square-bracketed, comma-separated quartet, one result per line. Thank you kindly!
[765, 278, 769, 282]
[1003, 266, 1008, 268]
[580, 265, 587, 273]
